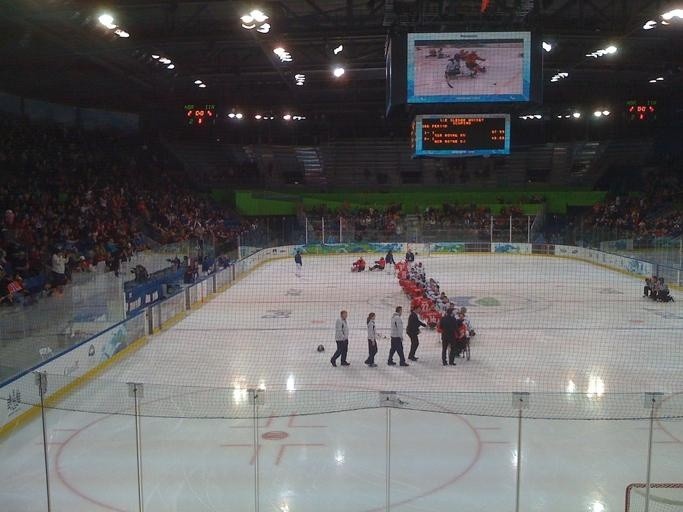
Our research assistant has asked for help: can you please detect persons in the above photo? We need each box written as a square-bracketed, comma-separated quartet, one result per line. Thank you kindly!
[330, 310, 350, 367]
[543, 146, 681, 246]
[2, 119, 269, 308]
[388, 306, 409, 365]
[353, 257, 365, 272]
[384, 250, 396, 270]
[439, 308, 458, 366]
[406, 305, 426, 360]
[304, 191, 543, 243]
[406, 249, 414, 262]
[365, 313, 378, 367]
[444, 47, 486, 79]
[295, 250, 302, 277]
[644, 276, 674, 302]
[395, 259, 475, 357]
[369, 257, 385, 271]
